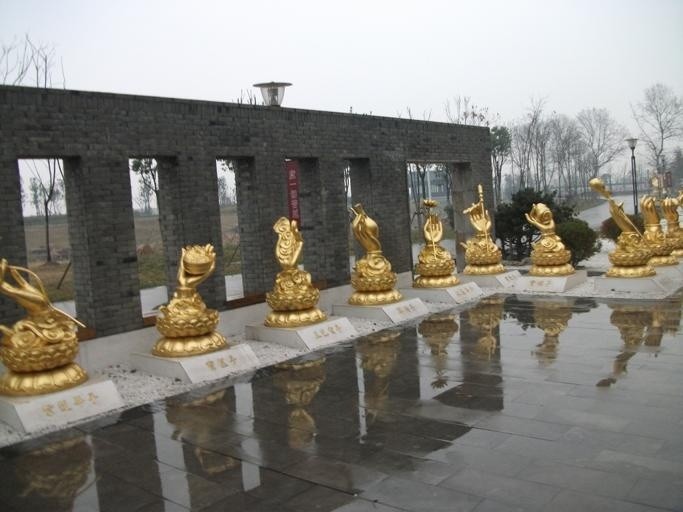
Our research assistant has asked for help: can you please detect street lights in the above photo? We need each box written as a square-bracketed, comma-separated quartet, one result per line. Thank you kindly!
[624, 135, 640, 215]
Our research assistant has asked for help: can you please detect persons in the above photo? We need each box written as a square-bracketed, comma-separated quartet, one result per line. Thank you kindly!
[475, 334, 497, 362]
[274, 220, 304, 270]
[524, 202, 556, 233]
[430, 346, 449, 376]
[467, 203, 492, 235]
[192, 427, 235, 475]
[607, 200, 634, 232]
[176, 242, 217, 288]
[644, 324, 663, 358]
[0, 258, 53, 316]
[610, 337, 640, 379]
[639, 194, 660, 224]
[423, 212, 443, 243]
[288, 408, 319, 455]
[661, 196, 679, 222]
[530, 329, 560, 369]
[352, 213, 381, 251]
